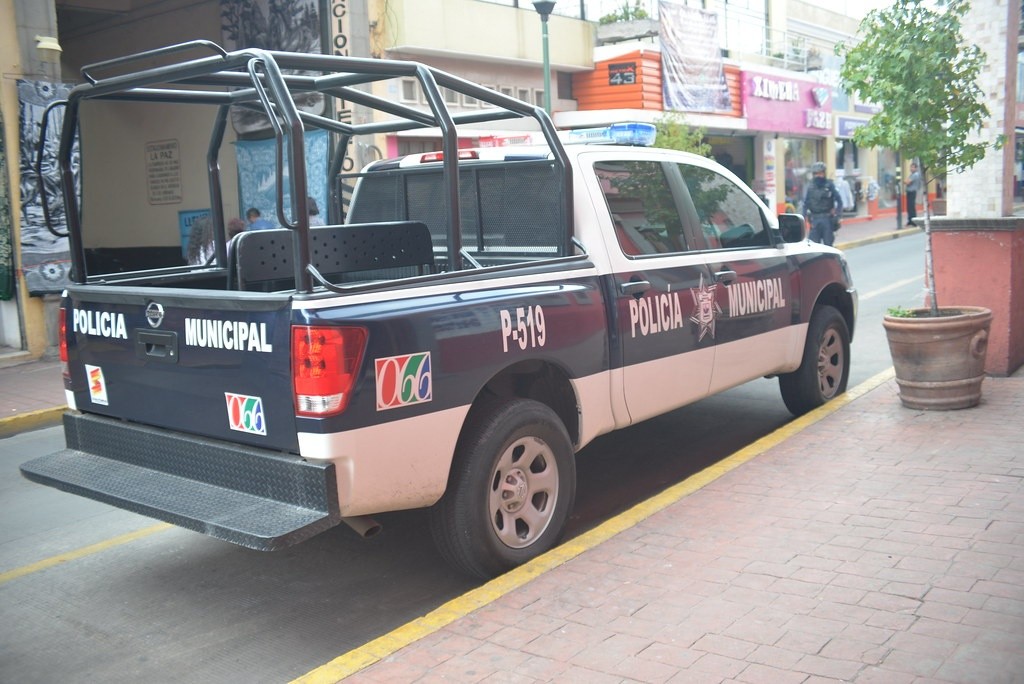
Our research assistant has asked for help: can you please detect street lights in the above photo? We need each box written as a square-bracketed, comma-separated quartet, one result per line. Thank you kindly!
[531, 0, 558, 118]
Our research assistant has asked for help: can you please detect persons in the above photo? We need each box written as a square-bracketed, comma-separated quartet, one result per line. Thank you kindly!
[184, 216, 218, 272]
[245, 208, 275, 230]
[225, 218, 248, 259]
[306, 196, 324, 227]
[900, 163, 920, 229]
[801, 162, 843, 247]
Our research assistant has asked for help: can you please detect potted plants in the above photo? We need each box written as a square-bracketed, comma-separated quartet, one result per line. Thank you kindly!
[837, 0, 1009, 410]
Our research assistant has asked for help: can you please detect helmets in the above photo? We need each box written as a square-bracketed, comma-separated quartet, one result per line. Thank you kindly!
[811, 163, 826, 187]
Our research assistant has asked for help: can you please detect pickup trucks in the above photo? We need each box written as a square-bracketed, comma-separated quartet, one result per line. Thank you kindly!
[17, 36, 877, 594]
